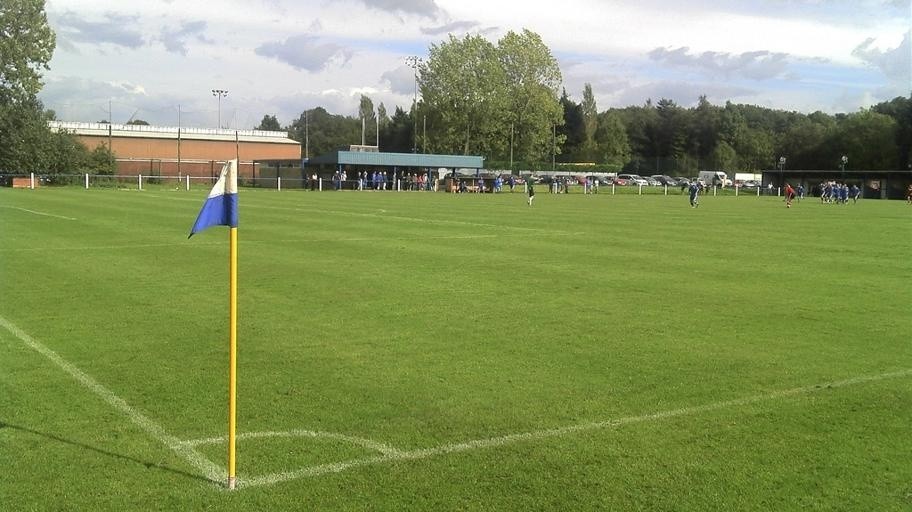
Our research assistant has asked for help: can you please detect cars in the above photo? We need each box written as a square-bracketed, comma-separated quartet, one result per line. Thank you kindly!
[445, 173, 499, 186]
[642, 176, 663, 187]
[732, 180, 761, 188]
[541, 174, 592, 186]
[605, 176, 627, 186]
[500, 174, 525, 185]
[522, 174, 540, 184]
[651, 175, 678, 187]
[617, 174, 648, 188]
[586, 175, 613, 187]
[674, 177, 708, 188]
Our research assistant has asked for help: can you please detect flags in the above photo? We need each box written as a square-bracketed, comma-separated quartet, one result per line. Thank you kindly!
[187, 160, 240, 241]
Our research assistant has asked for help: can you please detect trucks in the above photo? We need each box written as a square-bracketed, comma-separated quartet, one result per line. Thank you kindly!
[699, 171, 733, 186]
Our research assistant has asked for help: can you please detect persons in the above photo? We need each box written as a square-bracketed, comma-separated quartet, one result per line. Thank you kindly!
[311, 172, 318, 191]
[331, 170, 503, 194]
[584, 176, 599, 194]
[907, 184, 912, 203]
[547, 176, 569, 194]
[302, 173, 309, 191]
[767, 180, 861, 209]
[508, 176, 515, 193]
[681, 180, 705, 209]
[527, 174, 536, 206]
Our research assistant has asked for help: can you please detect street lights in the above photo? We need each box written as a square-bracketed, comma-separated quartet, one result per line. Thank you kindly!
[211, 89, 229, 128]
[404, 55, 423, 153]
